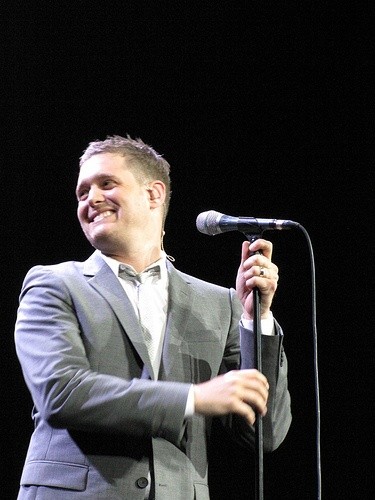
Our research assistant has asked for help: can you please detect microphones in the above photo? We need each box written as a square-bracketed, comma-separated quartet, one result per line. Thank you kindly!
[196, 210, 300, 235]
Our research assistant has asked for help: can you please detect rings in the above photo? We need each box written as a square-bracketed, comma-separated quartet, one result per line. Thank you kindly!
[260, 266, 265, 277]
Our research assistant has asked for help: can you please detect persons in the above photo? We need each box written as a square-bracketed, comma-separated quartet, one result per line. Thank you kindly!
[14, 136, 292, 500]
